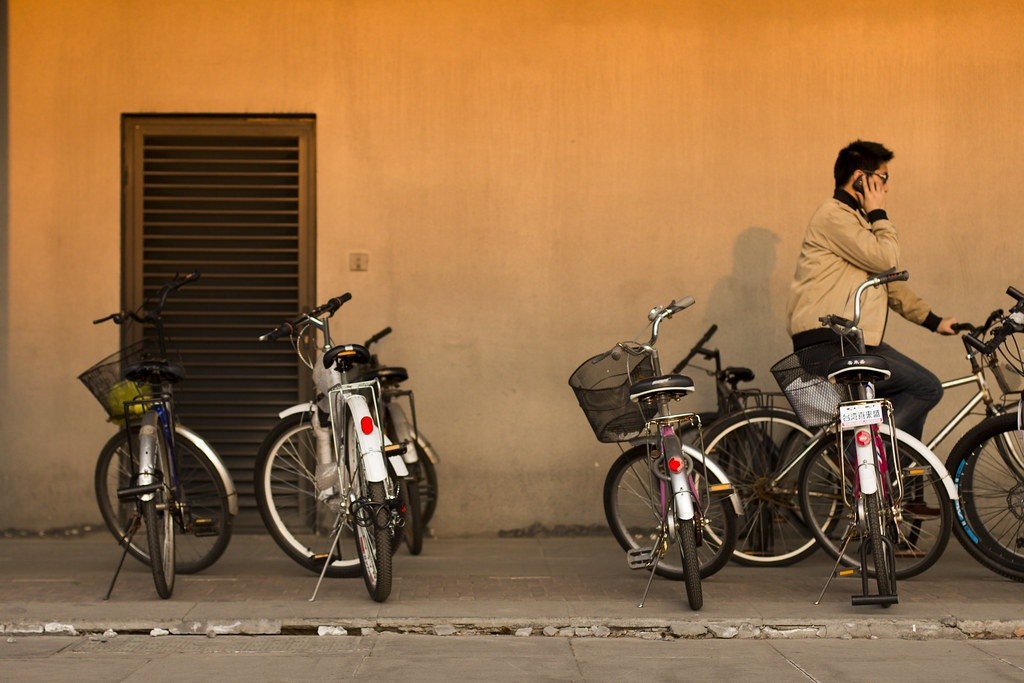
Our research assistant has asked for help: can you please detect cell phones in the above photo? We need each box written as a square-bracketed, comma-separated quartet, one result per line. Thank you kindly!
[853, 175, 870, 196]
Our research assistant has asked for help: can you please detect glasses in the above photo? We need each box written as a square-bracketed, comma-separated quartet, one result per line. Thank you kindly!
[863, 170, 888, 184]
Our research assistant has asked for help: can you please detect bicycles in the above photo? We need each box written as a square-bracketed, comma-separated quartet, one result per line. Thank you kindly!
[78, 270, 238, 601]
[670, 320, 788, 559]
[355, 326, 441, 556]
[694, 285, 1024, 583]
[569, 292, 745, 610]
[793, 267, 960, 610]
[252, 292, 410, 604]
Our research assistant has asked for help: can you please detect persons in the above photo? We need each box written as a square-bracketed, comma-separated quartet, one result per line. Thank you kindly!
[785, 140, 958, 518]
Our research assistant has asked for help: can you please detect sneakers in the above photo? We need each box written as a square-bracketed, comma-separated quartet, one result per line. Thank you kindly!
[903, 499, 942, 520]
